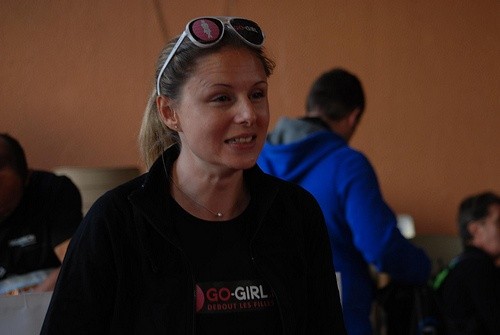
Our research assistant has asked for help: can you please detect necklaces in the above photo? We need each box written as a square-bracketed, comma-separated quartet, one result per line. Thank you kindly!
[174, 180, 238, 218]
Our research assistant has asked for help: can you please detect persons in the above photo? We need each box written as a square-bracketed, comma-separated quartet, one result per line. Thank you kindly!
[40, 15, 349, 335]
[428, 191, 499, 335]
[254, 67, 433, 335]
[0, 131, 82, 298]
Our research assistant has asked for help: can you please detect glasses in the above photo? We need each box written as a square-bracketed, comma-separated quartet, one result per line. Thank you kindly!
[157, 16, 266, 95]
